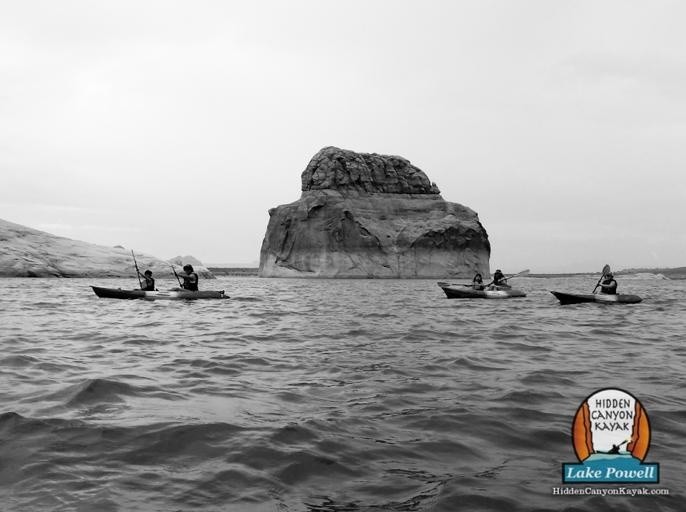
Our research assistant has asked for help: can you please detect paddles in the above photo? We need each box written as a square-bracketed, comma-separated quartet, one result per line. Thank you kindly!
[485, 269, 529, 287]
[437, 282, 483, 287]
[593, 265, 610, 294]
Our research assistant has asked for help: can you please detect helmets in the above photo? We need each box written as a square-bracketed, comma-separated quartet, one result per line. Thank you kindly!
[184, 265, 193, 272]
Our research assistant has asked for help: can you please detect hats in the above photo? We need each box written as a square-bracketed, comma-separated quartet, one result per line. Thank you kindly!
[605, 272, 613, 278]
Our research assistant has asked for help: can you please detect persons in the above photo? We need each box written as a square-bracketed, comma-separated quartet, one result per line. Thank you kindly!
[597, 273, 617, 294]
[136, 268, 154, 291]
[472, 270, 511, 291]
[177, 264, 198, 290]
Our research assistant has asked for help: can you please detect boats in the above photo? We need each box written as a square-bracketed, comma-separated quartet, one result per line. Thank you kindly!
[550, 290, 642, 306]
[441, 282, 526, 298]
[90, 285, 228, 301]
